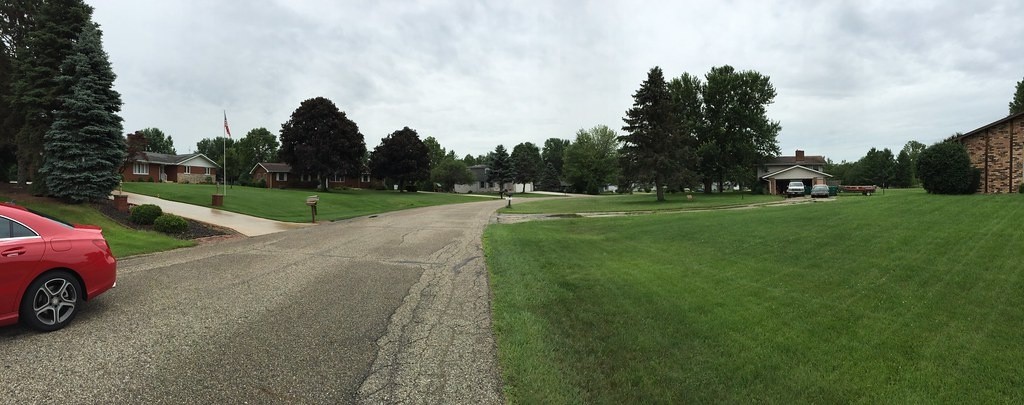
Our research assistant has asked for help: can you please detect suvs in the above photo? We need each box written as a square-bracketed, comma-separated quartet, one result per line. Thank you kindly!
[787, 181, 806, 198]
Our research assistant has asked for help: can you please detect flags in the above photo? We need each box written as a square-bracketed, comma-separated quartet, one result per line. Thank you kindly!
[225, 113, 232, 136]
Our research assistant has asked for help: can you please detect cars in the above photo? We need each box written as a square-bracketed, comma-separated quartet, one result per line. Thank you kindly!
[811, 184, 829, 199]
[1, 203, 118, 336]
